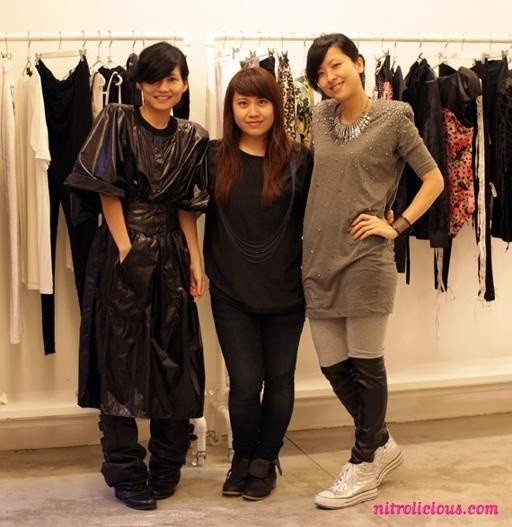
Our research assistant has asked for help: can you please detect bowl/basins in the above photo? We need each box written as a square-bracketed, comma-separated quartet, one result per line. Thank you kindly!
[326, 95, 372, 144]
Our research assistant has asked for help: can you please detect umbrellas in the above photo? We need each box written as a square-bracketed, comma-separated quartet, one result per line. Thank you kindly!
[115, 480, 178, 509]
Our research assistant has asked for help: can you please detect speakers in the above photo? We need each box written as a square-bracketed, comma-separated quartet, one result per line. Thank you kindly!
[222, 449, 282, 501]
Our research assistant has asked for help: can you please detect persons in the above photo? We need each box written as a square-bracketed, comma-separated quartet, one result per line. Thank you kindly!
[302, 33, 445, 511]
[65, 41, 211, 510]
[192, 66, 396, 500]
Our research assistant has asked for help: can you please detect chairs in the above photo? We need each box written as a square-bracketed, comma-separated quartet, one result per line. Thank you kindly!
[314, 460, 377, 509]
[375, 427, 403, 487]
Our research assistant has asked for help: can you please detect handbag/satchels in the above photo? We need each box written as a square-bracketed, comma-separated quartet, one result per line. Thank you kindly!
[389, 215, 411, 235]
